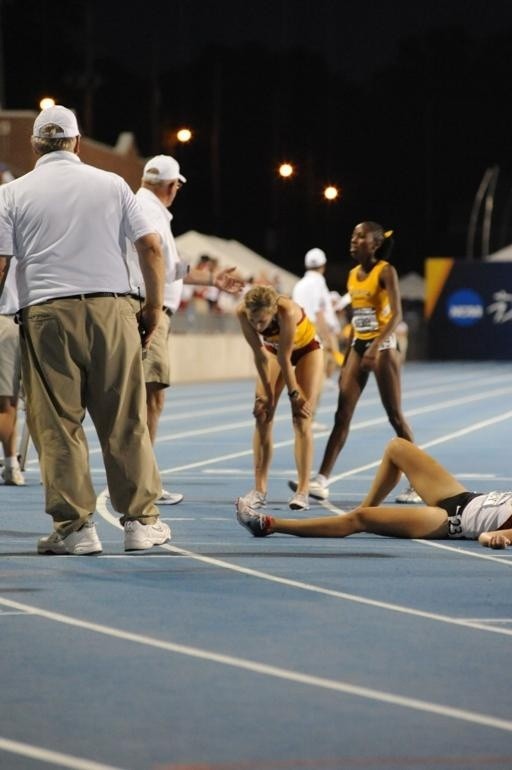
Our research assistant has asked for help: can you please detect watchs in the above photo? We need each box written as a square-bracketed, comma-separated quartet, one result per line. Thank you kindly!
[290, 390, 300, 399]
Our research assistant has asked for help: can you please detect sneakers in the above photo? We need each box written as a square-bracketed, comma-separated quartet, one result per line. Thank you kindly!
[244, 491, 269, 508]
[236, 498, 272, 538]
[123, 519, 171, 550]
[396, 486, 422, 503]
[155, 488, 183, 505]
[289, 479, 330, 503]
[5, 467, 25, 485]
[38, 521, 103, 556]
[290, 494, 310, 511]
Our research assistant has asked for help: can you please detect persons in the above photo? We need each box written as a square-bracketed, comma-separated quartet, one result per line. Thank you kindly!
[124, 155, 248, 506]
[0, 104, 172, 556]
[0, 255, 26, 487]
[290, 247, 343, 368]
[288, 220, 423, 504]
[235, 285, 326, 513]
[235, 436, 511, 551]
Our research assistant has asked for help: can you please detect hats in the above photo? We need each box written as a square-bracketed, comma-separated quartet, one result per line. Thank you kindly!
[33, 105, 79, 137]
[305, 248, 326, 268]
[144, 156, 186, 183]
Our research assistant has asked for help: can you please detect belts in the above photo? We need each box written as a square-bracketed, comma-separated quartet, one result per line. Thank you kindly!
[45, 292, 129, 299]
[131, 294, 174, 318]
